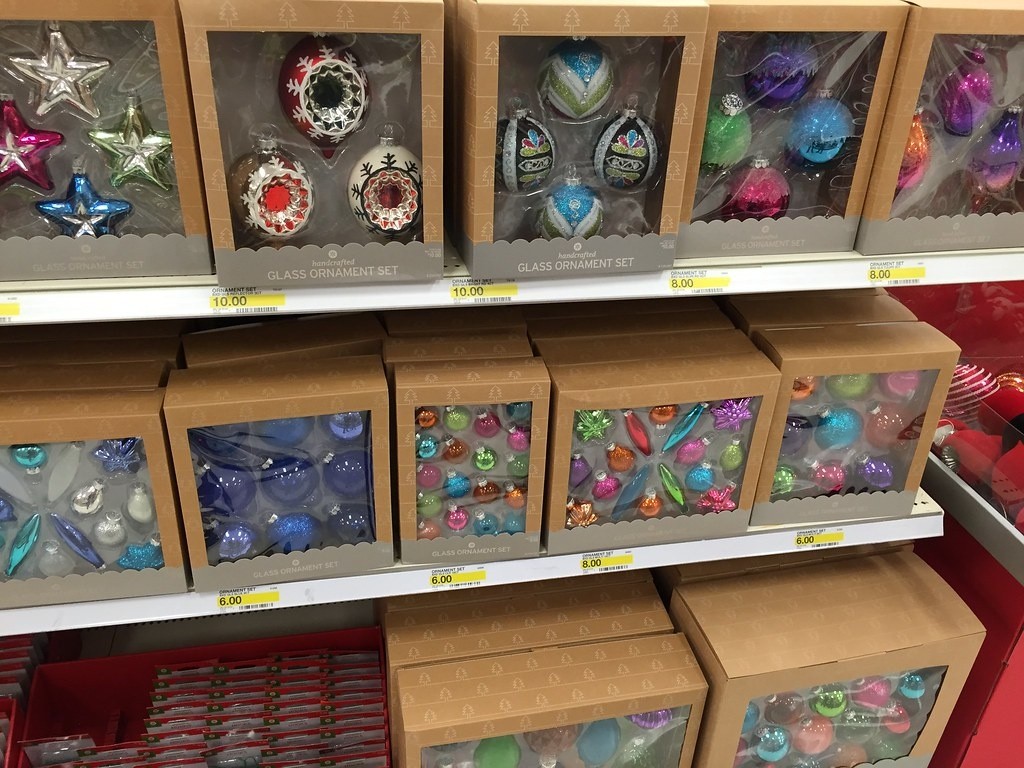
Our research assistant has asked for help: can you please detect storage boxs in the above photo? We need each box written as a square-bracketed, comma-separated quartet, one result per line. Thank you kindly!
[0, 0, 1024, 288]
[0, 289, 963, 608]
[0, 542, 987, 768]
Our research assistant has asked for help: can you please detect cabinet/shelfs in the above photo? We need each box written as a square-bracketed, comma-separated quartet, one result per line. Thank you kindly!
[0, 248, 1024, 639]
